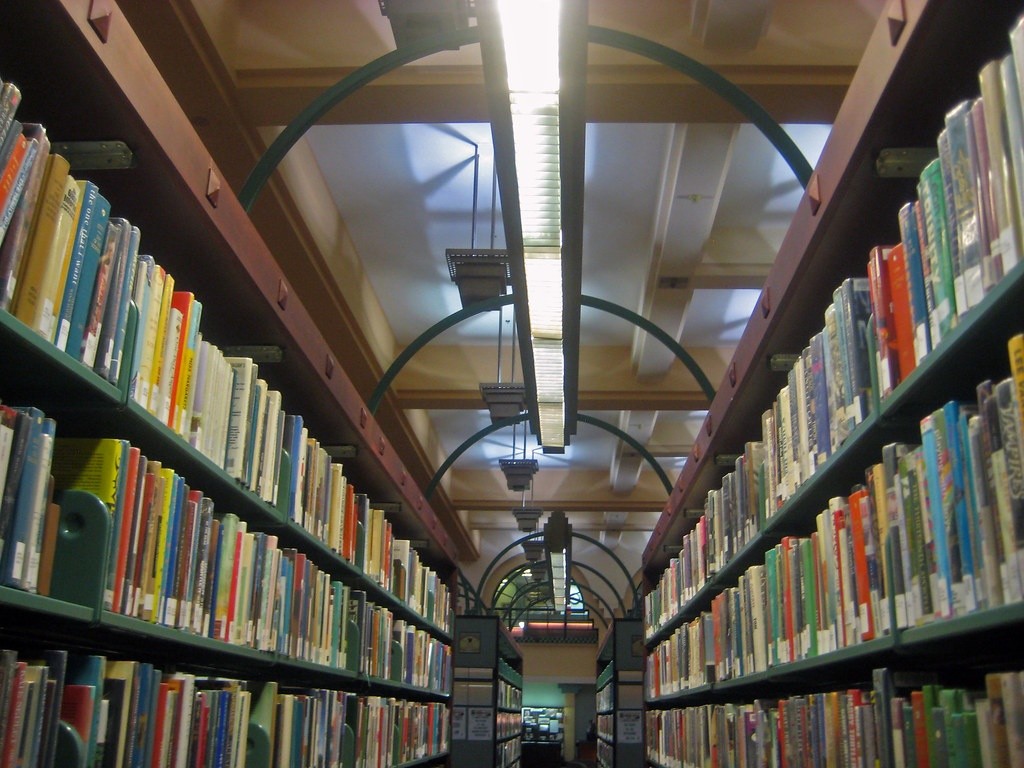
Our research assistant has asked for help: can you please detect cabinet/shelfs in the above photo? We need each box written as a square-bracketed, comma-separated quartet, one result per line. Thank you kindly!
[594, 612, 650, 767]
[451, 614, 524, 768]
[640, 1, 1022, 765]
[3, 0, 460, 768]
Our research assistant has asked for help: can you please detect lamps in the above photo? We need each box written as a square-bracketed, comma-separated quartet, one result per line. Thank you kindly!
[527, 554, 546, 582]
[475, 302, 530, 426]
[467, 0, 592, 452]
[542, 512, 573, 615]
[442, 145, 510, 317]
[520, 519, 547, 562]
[513, 449, 543, 534]
[499, 414, 539, 494]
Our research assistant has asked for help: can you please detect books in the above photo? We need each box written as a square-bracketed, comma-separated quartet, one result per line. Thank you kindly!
[645, 8, 1022, 767]
[498, 660, 522, 766]
[1, 78, 454, 765]
[597, 662, 613, 767]
